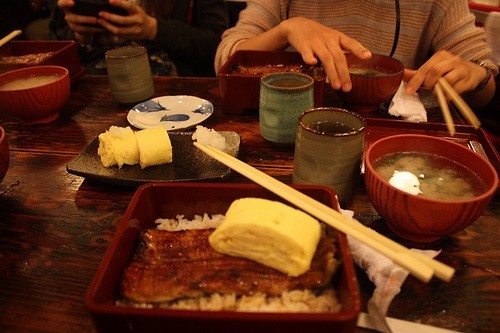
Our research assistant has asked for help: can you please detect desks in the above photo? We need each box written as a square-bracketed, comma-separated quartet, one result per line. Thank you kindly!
[0, 64, 500, 332]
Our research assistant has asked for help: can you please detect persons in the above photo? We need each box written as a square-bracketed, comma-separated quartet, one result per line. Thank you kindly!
[214, 0, 499, 102]
[49, 0, 229, 78]
[0, 0, 52, 41]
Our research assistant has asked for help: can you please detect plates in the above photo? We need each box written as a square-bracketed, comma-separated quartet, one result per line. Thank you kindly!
[127, 95, 214, 131]
[66, 131, 240, 184]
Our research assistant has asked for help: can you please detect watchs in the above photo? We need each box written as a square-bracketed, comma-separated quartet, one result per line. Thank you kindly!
[469, 59, 491, 94]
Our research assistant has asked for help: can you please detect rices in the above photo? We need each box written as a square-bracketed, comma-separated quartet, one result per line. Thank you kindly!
[114, 211, 343, 312]
[191, 125, 227, 151]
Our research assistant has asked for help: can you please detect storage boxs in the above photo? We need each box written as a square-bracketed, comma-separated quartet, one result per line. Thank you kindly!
[218, 49, 327, 118]
[85, 182, 361, 333]
[0, 39, 79, 77]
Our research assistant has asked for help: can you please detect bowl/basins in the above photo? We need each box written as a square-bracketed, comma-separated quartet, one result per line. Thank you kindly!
[0, 65, 71, 124]
[343, 52, 405, 112]
[84, 182, 362, 333]
[364, 133, 498, 243]
[217, 49, 326, 116]
[0, 40, 80, 77]
[0, 127, 10, 184]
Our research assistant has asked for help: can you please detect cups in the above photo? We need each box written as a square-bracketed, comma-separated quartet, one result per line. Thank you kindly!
[105, 45, 154, 103]
[259, 72, 314, 144]
[292, 107, 367, 197]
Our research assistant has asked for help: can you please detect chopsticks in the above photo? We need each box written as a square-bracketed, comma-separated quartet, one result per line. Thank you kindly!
[434, 77, 481, 136]
[0, 29, 22, 46]
[192, 141, 457, 284]
